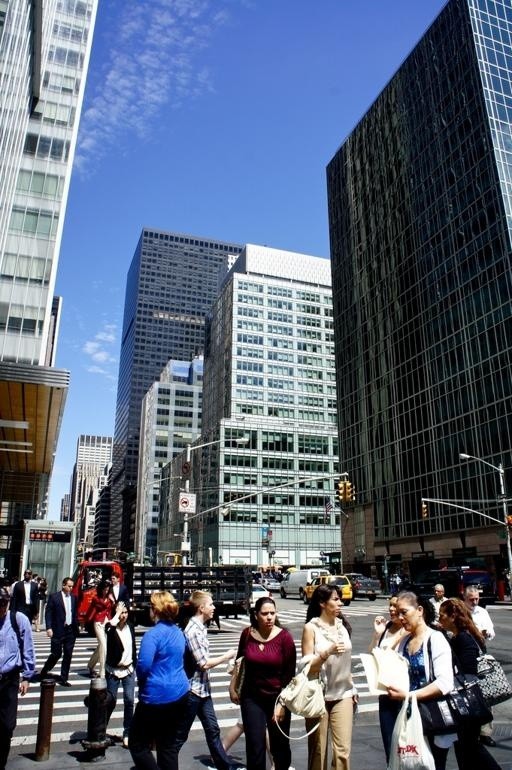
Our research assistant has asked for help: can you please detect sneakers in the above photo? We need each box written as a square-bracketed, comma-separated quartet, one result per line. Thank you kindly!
[123, 738, 129, 748]
[271, 763, 295, 770]
[88, 666, 97, 676]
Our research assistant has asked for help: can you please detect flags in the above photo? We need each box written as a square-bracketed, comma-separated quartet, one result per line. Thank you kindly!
[326, 502, 333, 512]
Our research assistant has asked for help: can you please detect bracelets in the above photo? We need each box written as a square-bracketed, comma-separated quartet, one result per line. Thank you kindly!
[319, 654, 326, 661]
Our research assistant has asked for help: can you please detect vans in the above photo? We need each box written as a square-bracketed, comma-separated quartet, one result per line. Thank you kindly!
[280, 569, 331, 599]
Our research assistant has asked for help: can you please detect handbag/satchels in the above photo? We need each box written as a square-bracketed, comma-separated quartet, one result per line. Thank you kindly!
[280, 660, 326, 718]
[453, 655, 511, 707]
[420, 676, 494, 736]
[229, 656, 245, 704]
[183, 645, 196, 677]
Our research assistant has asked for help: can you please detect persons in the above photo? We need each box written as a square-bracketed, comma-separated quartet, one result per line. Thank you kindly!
[129, 591, 192, 769]
[368, 568, 512, 770]
[106, 601, 137, 749]
[229, 597, 296, 770]
[0, 588, 35, 770]
[208, 706, 275, 770]
[0, 568, 129, 687]
[176, 590, 236, 769]
[302, 585, 358, 770]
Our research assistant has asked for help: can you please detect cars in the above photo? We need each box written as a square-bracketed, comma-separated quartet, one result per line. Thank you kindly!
[249, 570, 281, 609]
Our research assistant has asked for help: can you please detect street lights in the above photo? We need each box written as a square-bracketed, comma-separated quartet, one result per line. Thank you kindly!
[139, 476, 182, 565]
[459, 454, 512, 572]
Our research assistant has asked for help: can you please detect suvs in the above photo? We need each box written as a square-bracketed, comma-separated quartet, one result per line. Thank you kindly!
[400, 566, 497, 609]
[303, 575, 353, 606]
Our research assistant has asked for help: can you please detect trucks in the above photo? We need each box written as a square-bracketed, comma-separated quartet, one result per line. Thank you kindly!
[72, 560, 254, 636]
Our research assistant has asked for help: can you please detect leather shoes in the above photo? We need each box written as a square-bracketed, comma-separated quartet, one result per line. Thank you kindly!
[59, 681, 71, 687]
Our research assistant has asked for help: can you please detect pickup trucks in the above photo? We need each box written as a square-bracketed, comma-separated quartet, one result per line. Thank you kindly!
[339, 572, 382, 601]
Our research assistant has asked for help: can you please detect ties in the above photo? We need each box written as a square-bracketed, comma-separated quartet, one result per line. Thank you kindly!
[66, 595, 71, 625]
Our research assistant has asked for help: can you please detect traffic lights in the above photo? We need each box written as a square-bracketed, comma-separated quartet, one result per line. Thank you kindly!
[422, 504, 427, 518]
[336, 481, 346, 503]
[345, 482, 355, 502]
[182, 437, 249, 566]
